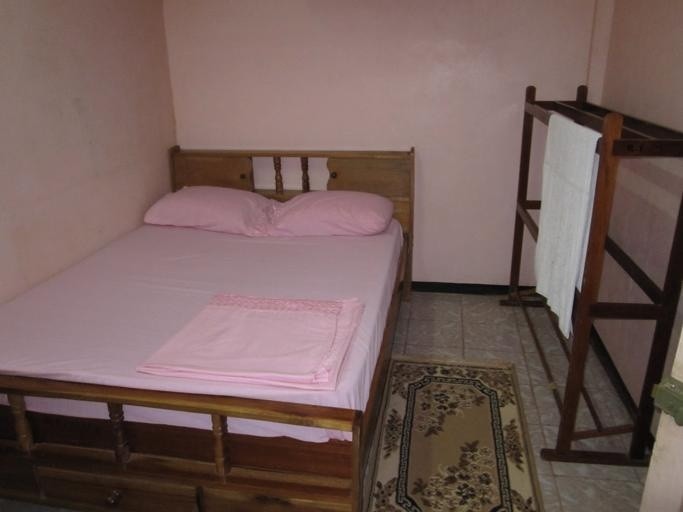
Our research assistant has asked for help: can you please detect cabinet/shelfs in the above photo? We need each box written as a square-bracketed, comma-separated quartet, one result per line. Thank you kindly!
[498, 82, 682, 468]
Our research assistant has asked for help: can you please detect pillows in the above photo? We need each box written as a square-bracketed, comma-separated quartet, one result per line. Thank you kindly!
[142, 184, 272, 236]
[273, 188, 396, 237]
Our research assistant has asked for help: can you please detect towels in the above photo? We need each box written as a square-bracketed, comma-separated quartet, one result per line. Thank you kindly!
[533, 112, 602, 340]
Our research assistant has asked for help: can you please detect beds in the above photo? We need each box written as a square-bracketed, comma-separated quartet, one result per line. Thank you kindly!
[0, 144, 415, 512]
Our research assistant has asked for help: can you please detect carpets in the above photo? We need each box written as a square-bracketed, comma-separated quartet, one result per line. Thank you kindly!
[357, 358, 542, 512]
